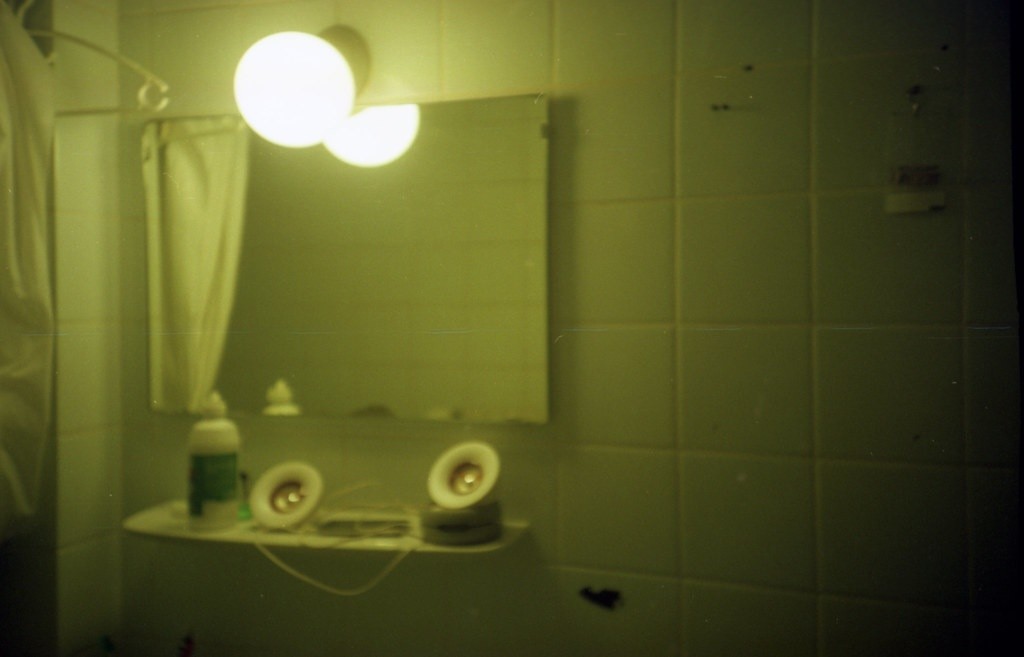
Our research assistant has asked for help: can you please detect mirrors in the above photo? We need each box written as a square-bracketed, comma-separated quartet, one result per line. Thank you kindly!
[138, 94, 552, 426]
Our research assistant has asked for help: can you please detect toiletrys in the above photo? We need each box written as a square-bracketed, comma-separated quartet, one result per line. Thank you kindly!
[263, 377, 301, 415]
[185, 391, 244, 519]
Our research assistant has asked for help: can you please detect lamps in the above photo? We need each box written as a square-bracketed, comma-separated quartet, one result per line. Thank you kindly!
[230, 25, 371, 151]
